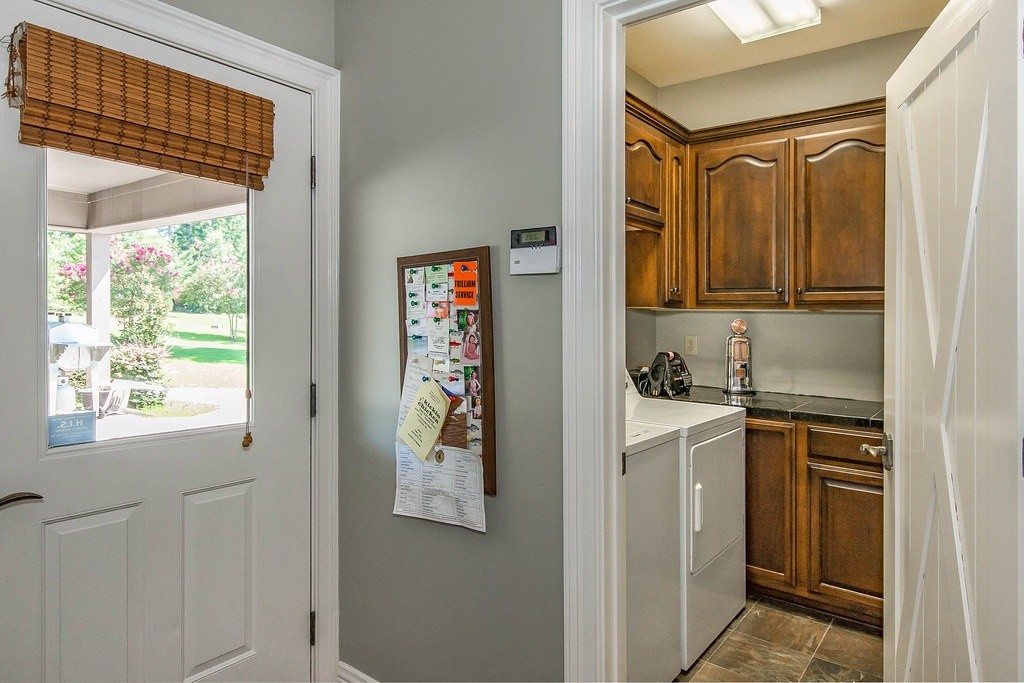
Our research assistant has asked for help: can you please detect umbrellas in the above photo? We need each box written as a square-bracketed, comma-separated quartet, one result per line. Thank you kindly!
[47, 321, 113, 372]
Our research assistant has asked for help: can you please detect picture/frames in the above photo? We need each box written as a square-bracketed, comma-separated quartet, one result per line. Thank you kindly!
[397, 244, 498, 496]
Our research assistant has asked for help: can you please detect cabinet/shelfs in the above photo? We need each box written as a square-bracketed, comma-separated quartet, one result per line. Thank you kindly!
[744, 417, 797, 605]
[625, 88, 691, 312]
[790, 412, 884, 635]
[685, 95, 885, 313]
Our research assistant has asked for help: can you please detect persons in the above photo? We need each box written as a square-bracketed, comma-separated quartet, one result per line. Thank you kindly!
[473, 398, 482, 418]
[469, 371, 481, 395]
[464, 334, 479, 359]
[466, 312, 476, 332]
